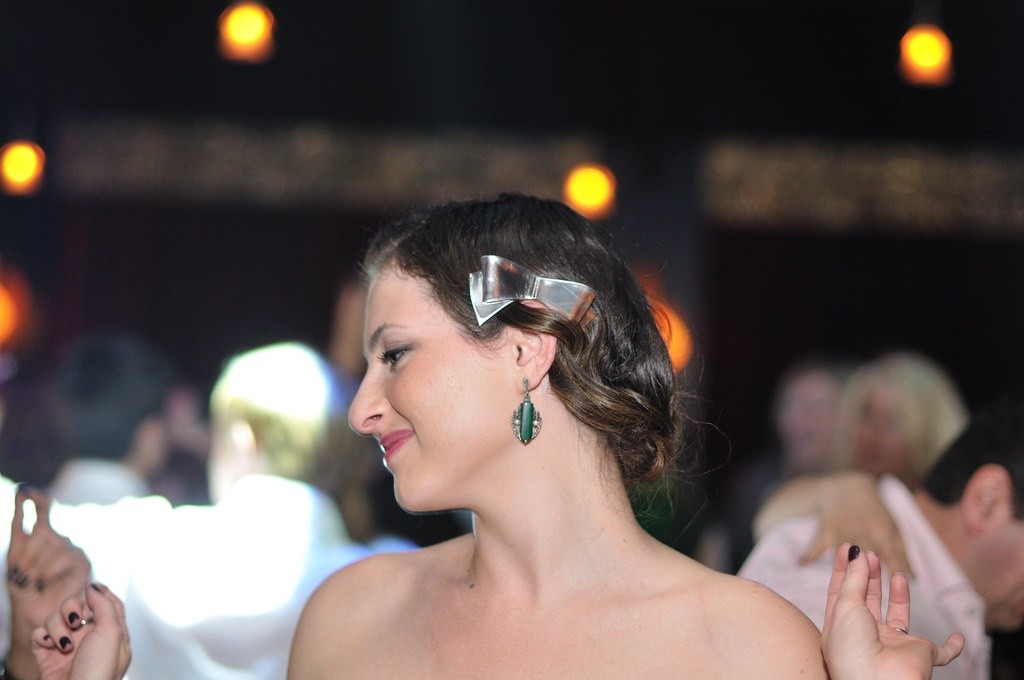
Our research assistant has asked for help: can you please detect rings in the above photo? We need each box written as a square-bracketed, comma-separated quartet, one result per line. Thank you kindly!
[80, 619, 92, 626]
[895, 628, 908, 635]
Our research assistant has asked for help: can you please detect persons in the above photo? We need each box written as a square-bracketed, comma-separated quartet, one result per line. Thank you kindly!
[0, 492, 91, 680]
[46, 377, 210, 510]
[697, 352, 1024, 680]
[29, 194, 966, 680]
[0, 343, 419, 680]
